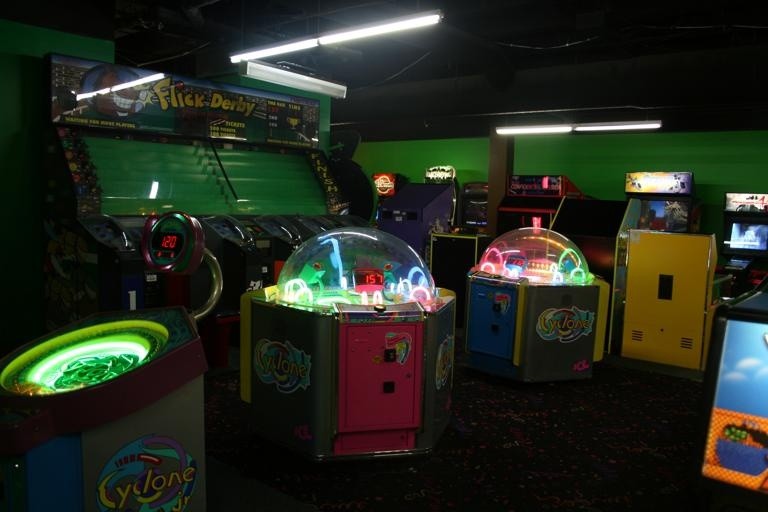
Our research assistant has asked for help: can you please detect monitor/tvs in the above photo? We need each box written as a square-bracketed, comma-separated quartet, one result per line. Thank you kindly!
[728, 220, 768, 252]
[638, 198, 688, 232]
